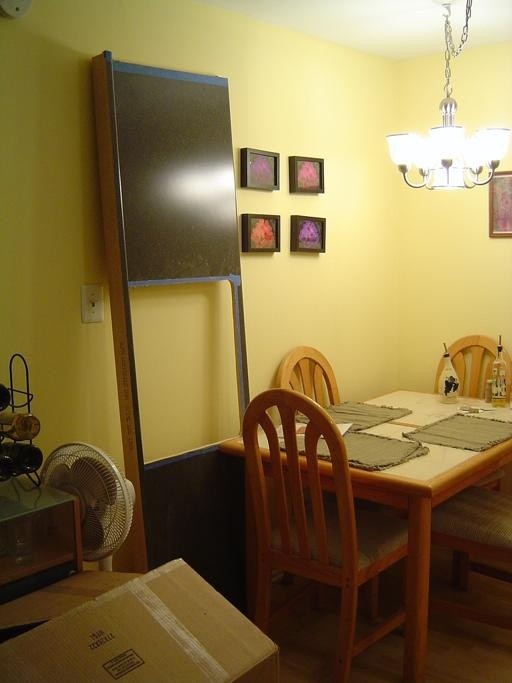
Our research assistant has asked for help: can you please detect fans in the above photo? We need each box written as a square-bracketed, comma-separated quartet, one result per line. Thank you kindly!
[40, 441, 137, 573]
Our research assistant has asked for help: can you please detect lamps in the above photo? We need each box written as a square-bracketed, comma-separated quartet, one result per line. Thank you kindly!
[386, 0, 512, 190]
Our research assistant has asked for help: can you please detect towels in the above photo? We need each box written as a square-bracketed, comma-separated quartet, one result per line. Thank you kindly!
[278, 400, 512, 472]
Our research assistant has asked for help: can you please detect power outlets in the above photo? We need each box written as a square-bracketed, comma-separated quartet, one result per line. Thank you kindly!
[80, 283, 105, 322]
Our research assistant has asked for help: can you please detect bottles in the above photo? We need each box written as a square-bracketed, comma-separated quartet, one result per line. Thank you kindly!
[0, 381, 44, 481]
[491, 334, 511, 408]
[438, 342, 460, 404]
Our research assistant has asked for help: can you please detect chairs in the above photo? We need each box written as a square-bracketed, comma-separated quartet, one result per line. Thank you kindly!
[434, 336, 510, 492]
[243, 389, 410, 680]
[275, 346, 340, 504]
[431, 486, 510, 647]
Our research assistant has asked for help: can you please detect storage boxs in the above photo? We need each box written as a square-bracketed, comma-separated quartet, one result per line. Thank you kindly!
[0, 557, 281, 683]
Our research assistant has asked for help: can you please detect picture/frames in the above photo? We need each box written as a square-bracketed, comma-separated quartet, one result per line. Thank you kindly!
[290, 215, 326, 252]
[288, 156, 324, 193]
[240, 148, 280, 190]
[241, 214, 280, 252]
[489, 171, 512, 237]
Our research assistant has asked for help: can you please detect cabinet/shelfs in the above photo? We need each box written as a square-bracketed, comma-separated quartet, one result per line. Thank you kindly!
[0, 476, 83, 607]
